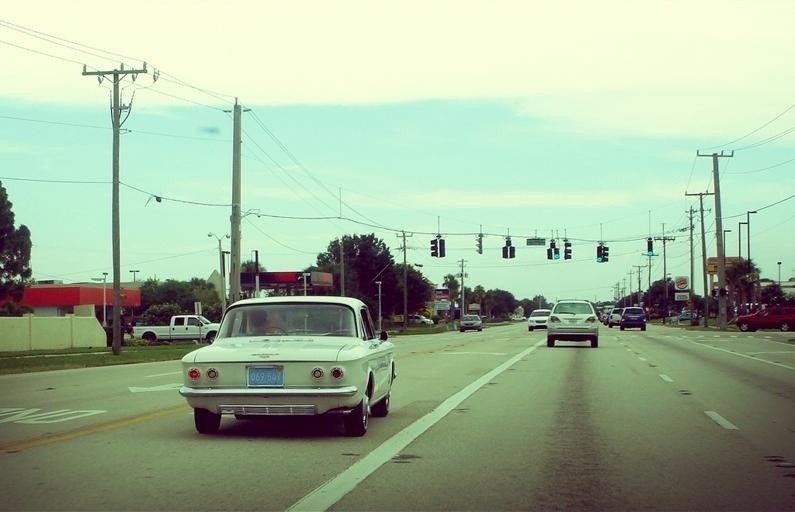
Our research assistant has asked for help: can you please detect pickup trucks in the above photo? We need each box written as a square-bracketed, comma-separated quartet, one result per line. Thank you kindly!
[130, 313, 222, 346]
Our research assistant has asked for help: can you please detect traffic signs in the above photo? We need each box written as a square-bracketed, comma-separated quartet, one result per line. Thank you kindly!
[527, 238, 545, 246]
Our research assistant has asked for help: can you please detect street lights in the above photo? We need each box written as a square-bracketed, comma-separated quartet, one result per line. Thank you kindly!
[129, 270, 140, 287]
[738, 222, 748, 261]
[207, 232, 231, 318]
[724, 229, 731, 265]
[747, 211, 758, 270]
[102, 272, 108, 327]
[374, 281, 382, 337]
[777, 261, 782, 286]
[302, 272, 311, 295]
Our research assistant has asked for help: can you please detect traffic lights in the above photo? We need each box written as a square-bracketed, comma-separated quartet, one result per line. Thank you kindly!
[509, 247, 515, 258]
[554, 248, 560, 260]
[548, 249, 552, 259]
[597, 246, 602, 262]
[507, 240, 512, 246]
[431, 239, 438, 257]
[565, 243, 571, 260]
[502, 247, 508, 258]
[476, 237, 483, 254]
[551, 242, 556, 248]
[603, 246, 609, 262]
[647, 240, 653, 256]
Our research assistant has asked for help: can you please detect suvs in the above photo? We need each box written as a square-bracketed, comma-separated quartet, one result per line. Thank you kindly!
[407, 314, 434, 325]
[736, 305, 795, 332]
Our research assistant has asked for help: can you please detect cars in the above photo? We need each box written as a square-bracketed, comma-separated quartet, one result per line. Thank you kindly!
[178, 296, 397, 437]
[460, 315, 483, 331]
[528, 298, 649, 348]
[678, 312, 702, 320]
